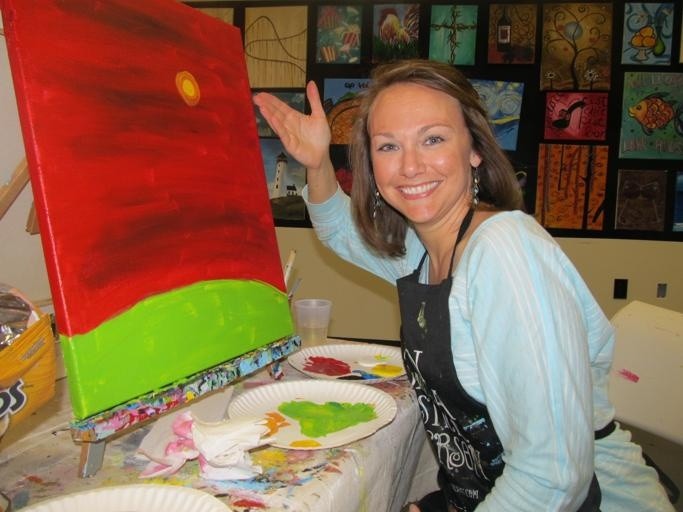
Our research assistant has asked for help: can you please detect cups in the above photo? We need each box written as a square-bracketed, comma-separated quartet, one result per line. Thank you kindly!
[293, 299, 331, 349]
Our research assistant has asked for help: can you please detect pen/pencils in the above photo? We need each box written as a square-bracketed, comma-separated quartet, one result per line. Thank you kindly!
[286, 277, 302, 301]
[283, 247, 299, 285]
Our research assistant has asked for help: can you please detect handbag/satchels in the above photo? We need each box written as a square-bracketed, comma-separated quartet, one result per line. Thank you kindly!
[0, 290, 57, 439]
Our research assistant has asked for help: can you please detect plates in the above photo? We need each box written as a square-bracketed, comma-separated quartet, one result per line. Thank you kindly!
[11, 483, 234, 512]
[286, 343, 407, 385]
[225, 377, 399, 452]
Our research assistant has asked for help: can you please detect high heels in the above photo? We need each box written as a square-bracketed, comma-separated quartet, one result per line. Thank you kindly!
[553, 101, 587, 129]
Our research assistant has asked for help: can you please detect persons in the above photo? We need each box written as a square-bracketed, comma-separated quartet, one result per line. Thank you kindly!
[249, 55, 678, 511]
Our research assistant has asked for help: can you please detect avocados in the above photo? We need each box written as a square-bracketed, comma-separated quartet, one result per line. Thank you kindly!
[653, 35, 665, 58]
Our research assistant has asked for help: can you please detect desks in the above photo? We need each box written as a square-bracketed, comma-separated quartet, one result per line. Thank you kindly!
[0, 336, 421, 511]
